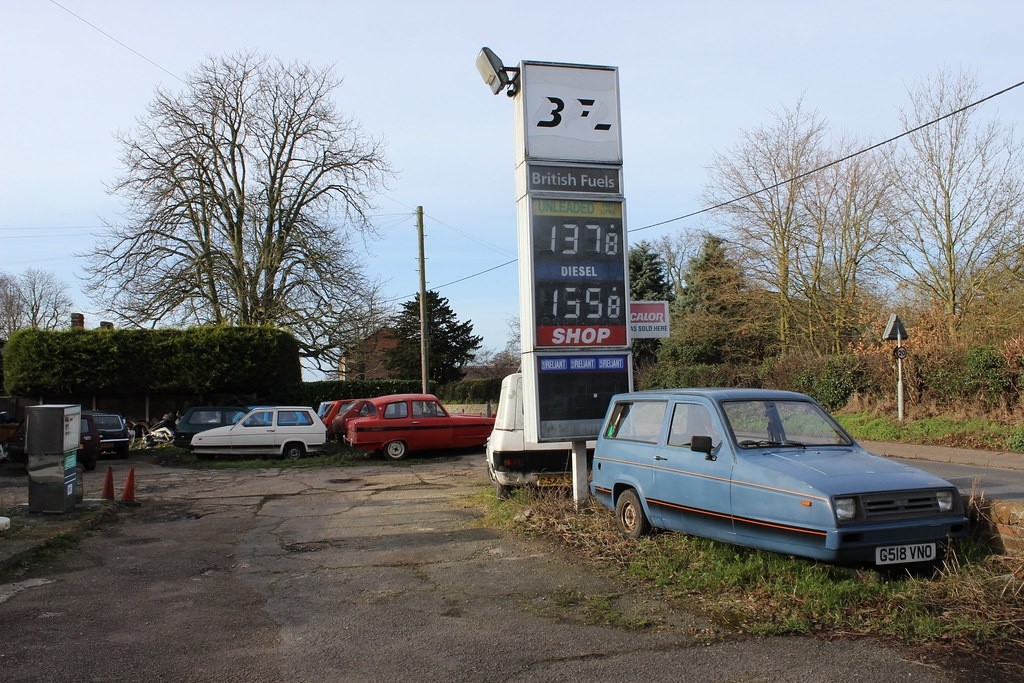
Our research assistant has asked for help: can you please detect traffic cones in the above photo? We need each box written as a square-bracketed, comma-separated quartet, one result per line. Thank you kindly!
[102, 466, 114, 500]
[121, 467, 135, 502]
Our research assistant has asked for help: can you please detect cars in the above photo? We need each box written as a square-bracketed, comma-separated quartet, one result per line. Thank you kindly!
[316, 402, 335, 417]
[173, 406, 265, 460]
[92, 412, 130, 456]
[321, 400, 352, 433]
[332, 399, 425, 443]
[483, 372, 597, 500]
[189, 405, 327, 460]
[590, 387, 969, 566]
[77, 415, 100, 471]
[232, 406, 309, 425]
[343, 394, 495, 461]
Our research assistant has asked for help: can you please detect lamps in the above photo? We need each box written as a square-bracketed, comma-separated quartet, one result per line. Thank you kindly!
[475, 46, 519, 96]
